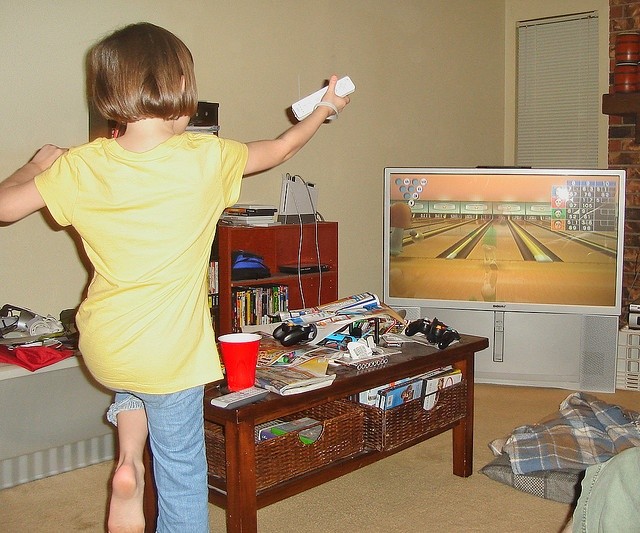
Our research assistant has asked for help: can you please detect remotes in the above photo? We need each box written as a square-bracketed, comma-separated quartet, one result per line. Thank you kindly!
[290, 74, 356, 122]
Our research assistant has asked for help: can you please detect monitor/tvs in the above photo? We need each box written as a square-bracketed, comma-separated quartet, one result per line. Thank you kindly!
[382, 165, 627, 318]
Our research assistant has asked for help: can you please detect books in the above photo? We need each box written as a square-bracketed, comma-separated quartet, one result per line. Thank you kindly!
[231, 286, 289, 334]
[358, 365, 463, 411]
[259, 417, 349, 462]
[205, 262, 218, 307]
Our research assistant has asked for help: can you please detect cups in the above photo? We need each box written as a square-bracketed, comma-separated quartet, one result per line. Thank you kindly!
[218, 333, 262, 391]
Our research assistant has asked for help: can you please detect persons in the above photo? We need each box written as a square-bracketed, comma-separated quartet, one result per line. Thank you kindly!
[0, 22, 349, 532]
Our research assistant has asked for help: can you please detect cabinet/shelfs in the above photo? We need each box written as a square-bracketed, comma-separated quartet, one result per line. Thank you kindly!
[207, 222, 338, 340]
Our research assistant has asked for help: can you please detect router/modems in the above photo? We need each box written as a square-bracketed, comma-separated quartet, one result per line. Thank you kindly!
[277, 178, 317, 224]
